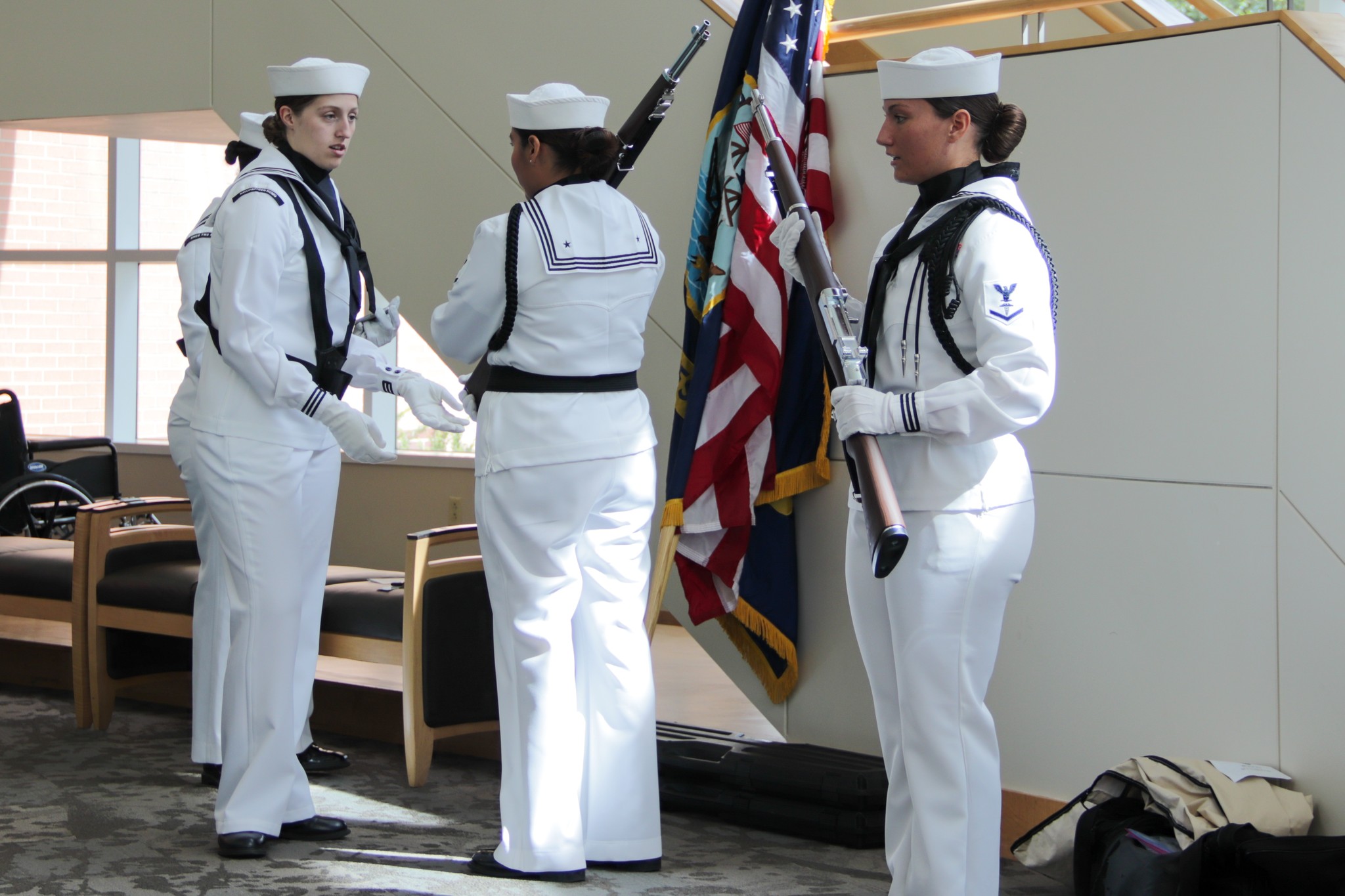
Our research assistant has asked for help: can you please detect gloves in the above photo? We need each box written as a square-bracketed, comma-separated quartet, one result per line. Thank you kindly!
[318, 394, 398, 468]
[354, 294, 403, 346]
[456, 371, 479, 421]
[395, 371, 470, 434]
[768, 208, 833, 290]
[829, 384, 896, 440]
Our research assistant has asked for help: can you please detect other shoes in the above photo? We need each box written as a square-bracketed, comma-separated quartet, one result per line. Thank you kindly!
[469, 847, 585, 884]
[585, 854, 665, 874]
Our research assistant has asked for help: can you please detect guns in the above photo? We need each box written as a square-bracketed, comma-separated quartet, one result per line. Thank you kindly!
[463, 18, 709, 411]
[746, 88, 910, 578]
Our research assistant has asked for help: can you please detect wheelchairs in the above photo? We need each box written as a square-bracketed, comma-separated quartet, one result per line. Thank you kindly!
[0, 389, 161, 542]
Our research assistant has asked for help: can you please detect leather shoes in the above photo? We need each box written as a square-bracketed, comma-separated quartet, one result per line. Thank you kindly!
[282, 814, 350, 840]
[295, 742, 351, 774]
[201, 762, 223, 784]
[218, 829, 269, 857]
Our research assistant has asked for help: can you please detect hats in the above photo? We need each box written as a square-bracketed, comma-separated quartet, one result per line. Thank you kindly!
[266, 57, 369, 100]
[238, 111, 275, 150]
[506, 82, 610, 131]
[877, 45, 1003, 102]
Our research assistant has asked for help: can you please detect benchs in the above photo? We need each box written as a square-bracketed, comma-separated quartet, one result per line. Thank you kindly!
[88, 498, 499, 787]
[1, 495, 177, 728]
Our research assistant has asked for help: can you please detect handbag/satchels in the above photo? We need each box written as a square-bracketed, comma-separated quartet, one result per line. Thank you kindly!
[1063, 783, 1345, 895]
